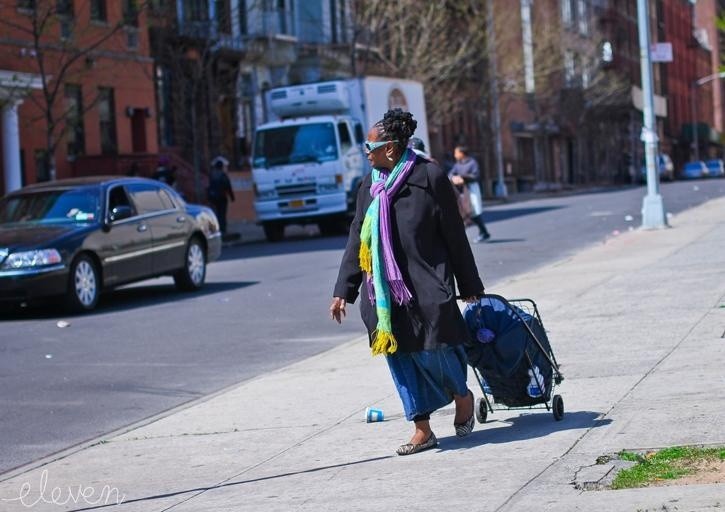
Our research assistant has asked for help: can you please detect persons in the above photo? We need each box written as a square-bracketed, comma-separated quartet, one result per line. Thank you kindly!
[209, 160, 235, 236]
[447, 146, 490, 242]
[329, 108, 485, 456]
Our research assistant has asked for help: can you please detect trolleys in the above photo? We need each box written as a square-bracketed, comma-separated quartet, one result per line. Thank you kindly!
[445, 293, 567, 426]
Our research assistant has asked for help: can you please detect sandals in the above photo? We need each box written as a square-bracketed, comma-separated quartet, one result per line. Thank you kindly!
[396, 432, 439, 456]
[453, 390, 476, 438]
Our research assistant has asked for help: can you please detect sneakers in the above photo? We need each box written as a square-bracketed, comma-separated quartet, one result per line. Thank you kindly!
[475, 233, 489, 243]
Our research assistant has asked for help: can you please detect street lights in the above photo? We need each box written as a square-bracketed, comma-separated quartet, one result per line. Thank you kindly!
[690, 70, 725, 162]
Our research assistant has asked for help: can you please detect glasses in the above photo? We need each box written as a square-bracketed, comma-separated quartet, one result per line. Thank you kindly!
[363, 139, 397, 155]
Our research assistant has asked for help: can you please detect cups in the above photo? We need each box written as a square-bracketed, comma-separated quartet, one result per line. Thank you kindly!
[365, 407, 384, 423]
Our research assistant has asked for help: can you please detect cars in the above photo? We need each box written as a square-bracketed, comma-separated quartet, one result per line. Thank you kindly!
[1, 169, 224, 314]
[638, 152, 725, 184]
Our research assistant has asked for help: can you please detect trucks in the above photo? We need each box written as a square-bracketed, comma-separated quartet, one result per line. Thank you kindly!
[247, 75, 434, 240]
